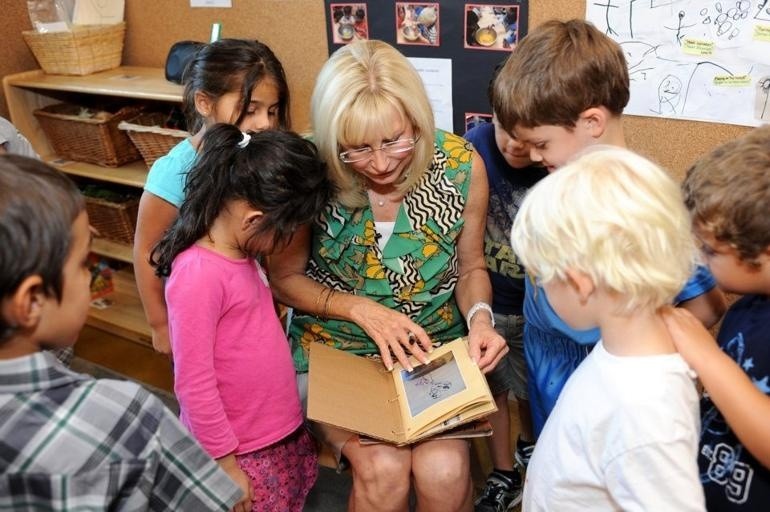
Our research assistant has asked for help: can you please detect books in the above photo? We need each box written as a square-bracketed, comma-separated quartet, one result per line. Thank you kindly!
[306, 338, 499, 447]
[87, 251, 114, 301]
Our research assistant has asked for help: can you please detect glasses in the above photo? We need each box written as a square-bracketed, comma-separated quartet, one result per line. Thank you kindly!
[338, 129, 420, 165]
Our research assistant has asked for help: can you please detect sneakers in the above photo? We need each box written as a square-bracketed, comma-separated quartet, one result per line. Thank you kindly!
[473, 464, 522, 511]
[513, 434, 535, 469]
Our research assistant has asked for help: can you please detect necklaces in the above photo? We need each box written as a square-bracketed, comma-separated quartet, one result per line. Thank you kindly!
[370, 181, 394, 209]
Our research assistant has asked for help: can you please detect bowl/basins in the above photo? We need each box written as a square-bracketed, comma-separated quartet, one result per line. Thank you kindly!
[337, 25, 354, 40]
[402, 25, 421, 41]
[475, 27, 498, 47]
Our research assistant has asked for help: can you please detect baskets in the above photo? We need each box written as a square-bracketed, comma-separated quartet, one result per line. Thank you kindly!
[119, 110, 187, 173]
[24, 23, 125, 75]
[82, 189, 137, 247]
[33, 100, 139, 164]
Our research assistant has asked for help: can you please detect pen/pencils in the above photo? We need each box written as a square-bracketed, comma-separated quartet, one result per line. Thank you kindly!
[408, 330, 416, 345]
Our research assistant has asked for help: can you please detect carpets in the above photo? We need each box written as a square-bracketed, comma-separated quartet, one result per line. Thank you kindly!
[64, 358, 489, 512]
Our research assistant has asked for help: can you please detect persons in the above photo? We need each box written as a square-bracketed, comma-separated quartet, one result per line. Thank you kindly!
[466, 5, 518, 50]
[458, 55, 551, 511]
[148, 122, 334, 510]
[331, 4, 369, 39]
[492, 18, 727, 445]
[267, 37, 511, 511]
[510, 146, 708, 511]
[132, 37, 289, 357]
[654, 122, 769, 512]
[397, 4, 438, 45]
[0, 152, 245, 511]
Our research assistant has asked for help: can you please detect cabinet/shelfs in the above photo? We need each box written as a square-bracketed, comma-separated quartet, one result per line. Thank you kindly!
[1, 63, 186, 355]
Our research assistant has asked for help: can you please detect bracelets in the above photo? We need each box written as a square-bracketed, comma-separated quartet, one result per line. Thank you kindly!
[465, 298, 497, 332]
[316, 285, 341, 323]
